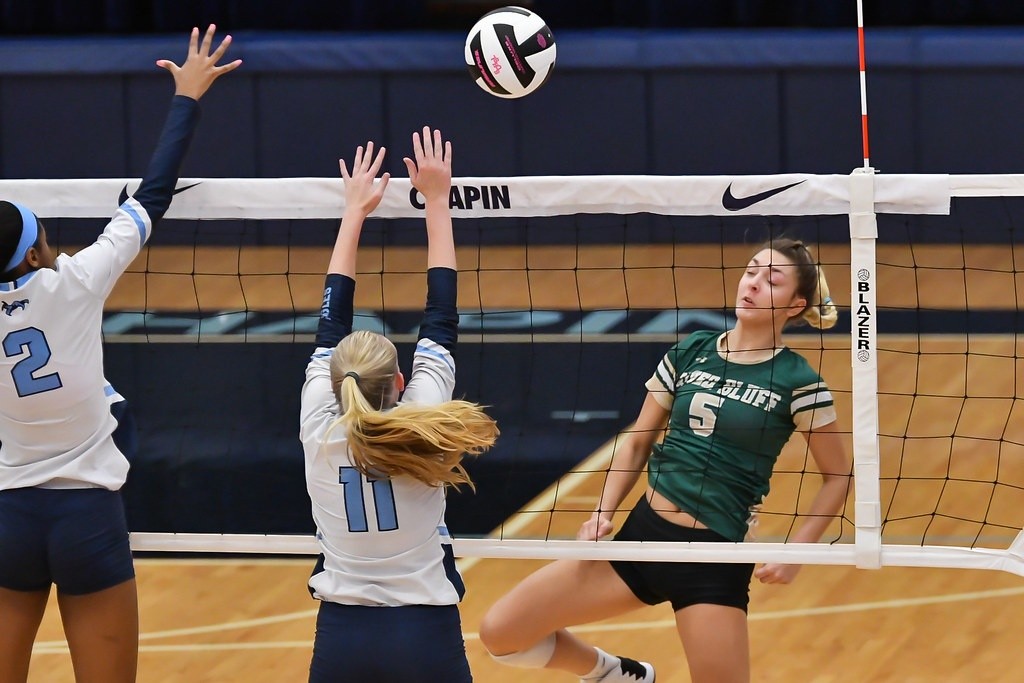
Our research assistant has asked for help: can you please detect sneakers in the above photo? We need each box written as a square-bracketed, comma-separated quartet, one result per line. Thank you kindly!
[578, 656, 656, 683]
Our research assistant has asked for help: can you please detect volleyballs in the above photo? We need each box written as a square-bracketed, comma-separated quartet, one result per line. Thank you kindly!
[464, 6, 557, 101]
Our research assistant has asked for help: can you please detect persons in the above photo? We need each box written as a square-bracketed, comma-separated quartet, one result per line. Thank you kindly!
[478, 238, 851, 683]
[0, 24, 242, 683]
[298, 127, 474, 683]
